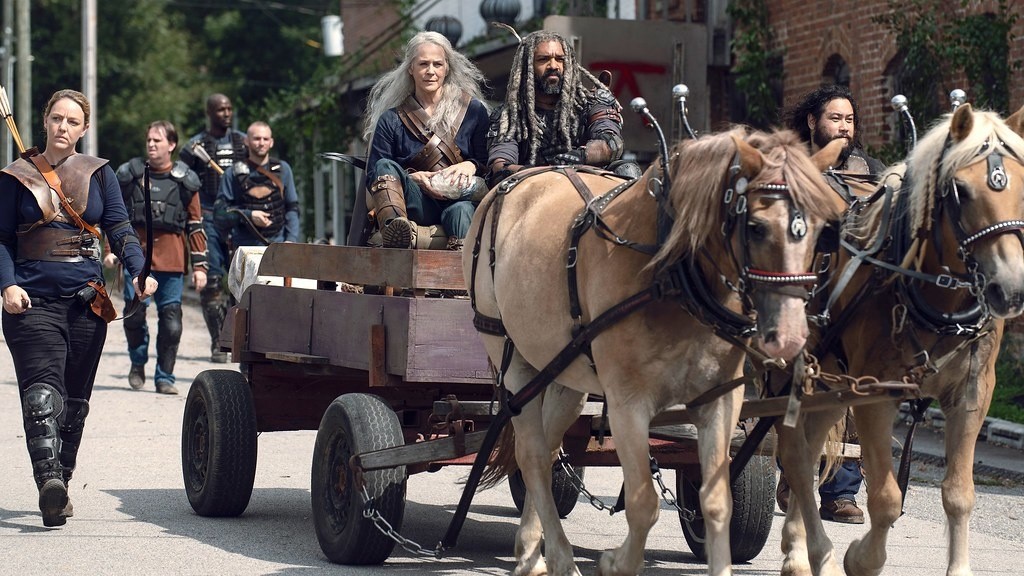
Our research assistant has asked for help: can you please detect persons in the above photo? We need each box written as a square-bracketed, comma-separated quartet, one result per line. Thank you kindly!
[212, 119, 299, 264]
[179, 90, 250, 363]
[358, 30, 495, 249]
[772, 84, 891, 526]
[0, 88, 158, 530]
[481, 27, 631, 177]
[112, 119, 210, 396]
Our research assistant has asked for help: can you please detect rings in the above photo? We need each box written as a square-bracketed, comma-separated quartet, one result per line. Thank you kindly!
[461, 174, 468, 177]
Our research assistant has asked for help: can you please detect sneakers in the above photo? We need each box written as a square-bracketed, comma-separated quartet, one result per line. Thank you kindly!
[157, 382, 177, 394]
[775, 469, 788, 513]
[37, 479, 74, 526]
[818, 499, 865, 524]
[128, 365, 145, 390]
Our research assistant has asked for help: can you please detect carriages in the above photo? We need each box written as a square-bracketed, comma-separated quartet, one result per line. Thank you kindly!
[181, 83, 1024, 576]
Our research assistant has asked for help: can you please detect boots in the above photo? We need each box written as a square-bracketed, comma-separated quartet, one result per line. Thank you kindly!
[440, 232, 467, 297]
[369, 176, 412, 249]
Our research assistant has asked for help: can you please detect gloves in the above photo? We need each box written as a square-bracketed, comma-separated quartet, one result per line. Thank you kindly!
[549, 149, 584, 166]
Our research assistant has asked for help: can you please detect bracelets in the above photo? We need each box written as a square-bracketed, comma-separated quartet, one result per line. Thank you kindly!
[500, 160, 514, 172]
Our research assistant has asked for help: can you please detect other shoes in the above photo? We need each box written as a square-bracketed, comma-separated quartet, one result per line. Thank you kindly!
[211, 349, 227, 363]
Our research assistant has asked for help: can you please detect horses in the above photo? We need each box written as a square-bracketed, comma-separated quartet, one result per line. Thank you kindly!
[462, 124, 833, 576]
[745, 104, 1024, 576]
